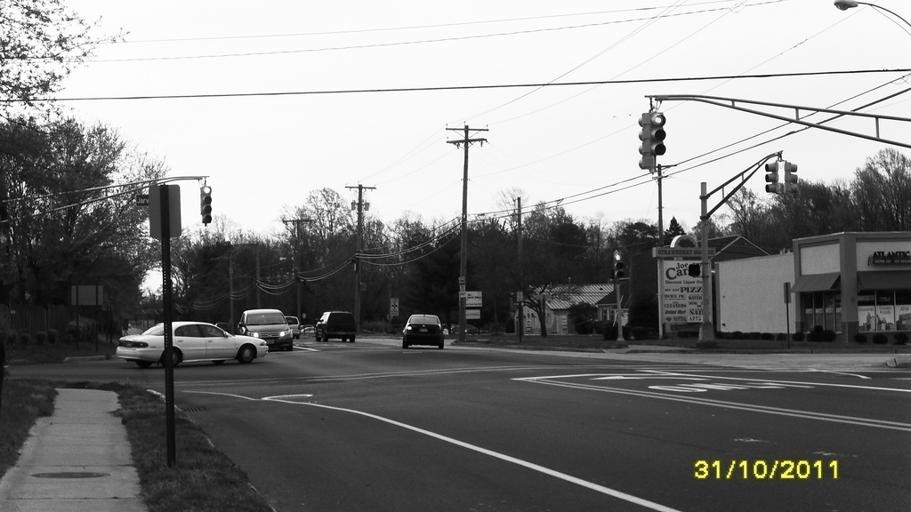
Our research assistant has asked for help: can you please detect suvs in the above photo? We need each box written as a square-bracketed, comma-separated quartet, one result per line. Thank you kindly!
[312, 310, 357, 343]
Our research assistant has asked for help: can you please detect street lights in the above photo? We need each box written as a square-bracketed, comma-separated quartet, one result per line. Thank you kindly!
[831, 0, 910, 26]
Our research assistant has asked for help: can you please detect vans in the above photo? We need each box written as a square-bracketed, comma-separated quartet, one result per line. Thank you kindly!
[283, 313, 301, 340]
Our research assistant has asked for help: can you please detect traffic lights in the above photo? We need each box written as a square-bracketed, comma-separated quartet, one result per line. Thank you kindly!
[783, 158, 799, 194]
[688, 263, 702, 278]
[637, 111, 655, 171]
[643, 109, 666, 156]
[611, 248, 625, 285]
[199, 185, 213, 225]
[763, 160, 779, 194]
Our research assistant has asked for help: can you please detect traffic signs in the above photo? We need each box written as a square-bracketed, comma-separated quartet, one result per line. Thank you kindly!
[136, 192, 149, 207]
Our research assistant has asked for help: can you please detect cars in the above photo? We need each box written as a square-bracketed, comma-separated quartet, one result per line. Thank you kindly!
[209, 322, 231, 334]
[400, 312, 446, 350]
[446, 322, 478, 336]
[114, 317, 269, 369]
[299, 324, 316, 335]
[235, 308, 295, 352]
